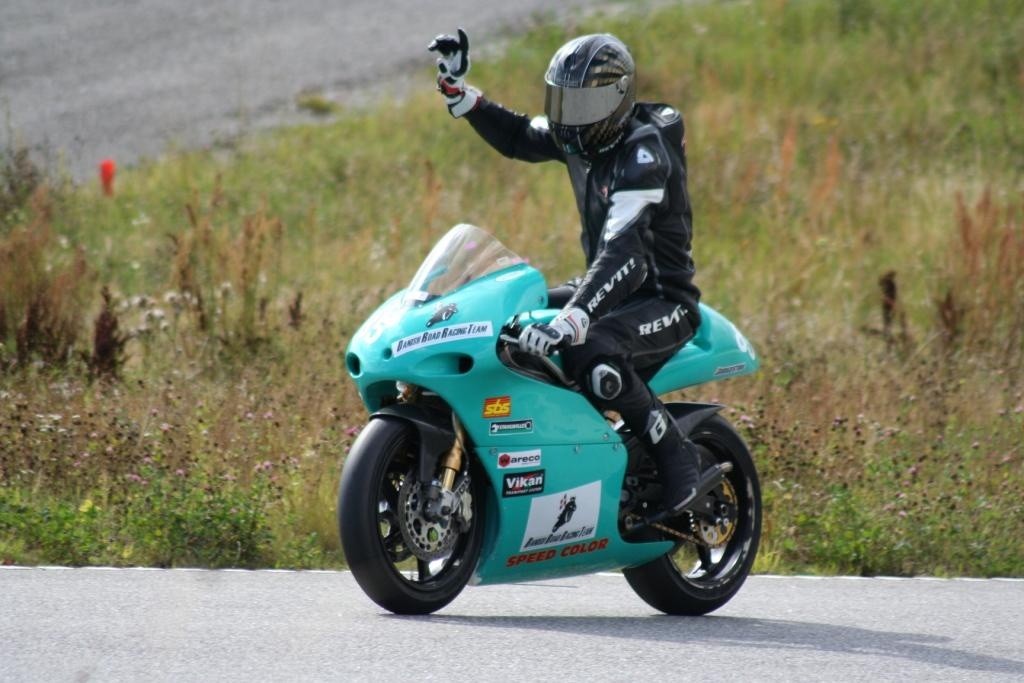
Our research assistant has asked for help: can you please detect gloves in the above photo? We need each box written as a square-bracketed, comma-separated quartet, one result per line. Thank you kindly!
[519, 308, 590, 359]
[428, 28, 470, 99]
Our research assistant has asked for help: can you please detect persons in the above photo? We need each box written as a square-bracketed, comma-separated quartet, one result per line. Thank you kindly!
[426, 26, 701, 514]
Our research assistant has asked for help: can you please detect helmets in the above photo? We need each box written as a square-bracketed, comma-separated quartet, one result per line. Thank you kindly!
[543, 33, 637, 155]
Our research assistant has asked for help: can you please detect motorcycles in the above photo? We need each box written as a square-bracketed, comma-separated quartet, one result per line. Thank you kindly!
[330, 223, 766, 619]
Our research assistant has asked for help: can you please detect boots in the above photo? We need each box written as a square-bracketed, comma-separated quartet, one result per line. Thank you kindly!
[627, 401, 701, 516]
